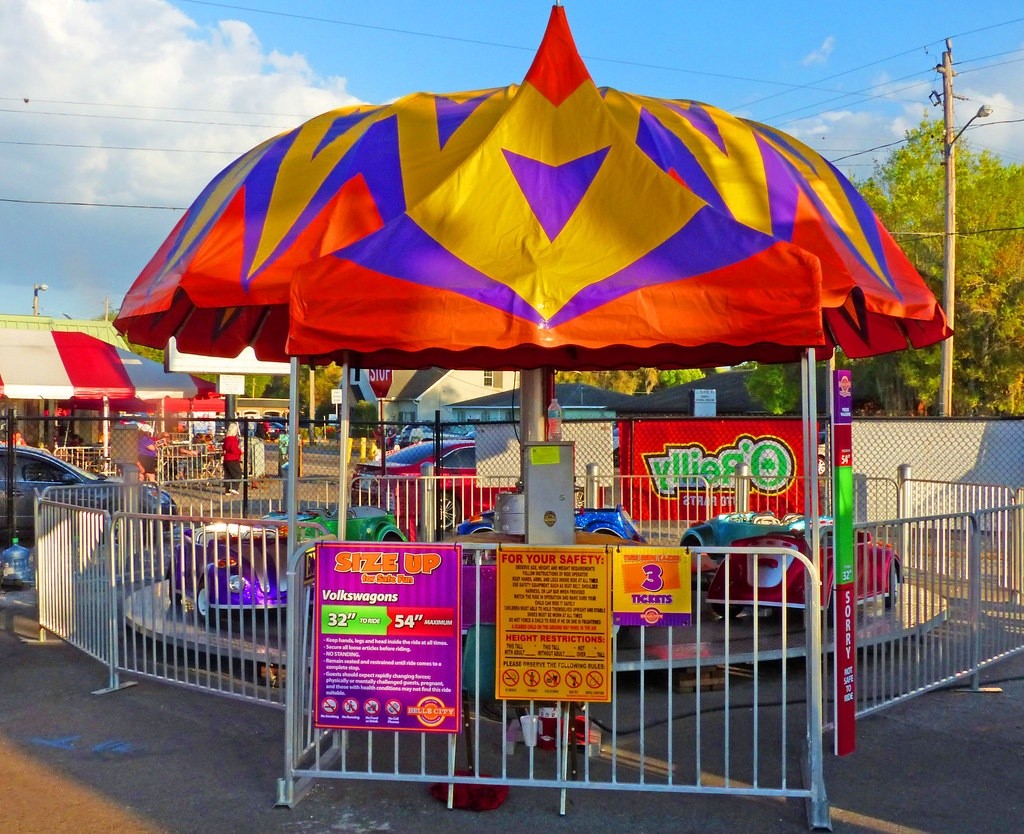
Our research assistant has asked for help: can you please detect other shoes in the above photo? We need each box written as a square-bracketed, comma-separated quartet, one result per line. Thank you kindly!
[229, 489, 239, 494]
[225, 492, 231, 496]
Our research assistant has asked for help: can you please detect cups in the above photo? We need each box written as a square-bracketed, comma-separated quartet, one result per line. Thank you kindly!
[521, 715, 538, 747]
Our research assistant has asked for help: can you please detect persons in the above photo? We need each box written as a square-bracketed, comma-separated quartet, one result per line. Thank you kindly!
[160, 431, 217, 458]
[10, 431, 30, 447]
[222, 423, 245, 497]
[138, 424, 156, 482]
[333, 424, 396, 451]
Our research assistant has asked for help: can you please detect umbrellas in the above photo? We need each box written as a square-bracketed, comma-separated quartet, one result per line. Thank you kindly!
[109, 7, 952, 493]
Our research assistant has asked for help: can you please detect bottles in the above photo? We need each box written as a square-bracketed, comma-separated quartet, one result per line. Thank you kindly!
[547, 399, 562, 440]
[589, 720, 601, 757]
[2, 538, 30, 581]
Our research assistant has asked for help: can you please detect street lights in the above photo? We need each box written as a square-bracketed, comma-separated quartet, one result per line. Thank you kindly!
[34, 281, 49, 317]
[939, 104, 996, 420]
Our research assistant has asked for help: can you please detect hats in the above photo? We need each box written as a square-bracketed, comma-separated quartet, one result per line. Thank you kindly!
[138, 424, 154, 433]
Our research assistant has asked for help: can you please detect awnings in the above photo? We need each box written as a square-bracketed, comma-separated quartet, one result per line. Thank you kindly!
[0, 329, 235, 444]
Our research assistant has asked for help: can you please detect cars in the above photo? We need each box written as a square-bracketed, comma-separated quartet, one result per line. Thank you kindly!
[237, 411, 289, 440]
[0, 445, 178, 545]
[165, 505, 906, 646]
[351, 438, 525, 542]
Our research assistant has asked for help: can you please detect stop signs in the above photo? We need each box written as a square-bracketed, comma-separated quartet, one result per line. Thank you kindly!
[368, 369, 392, 397]
